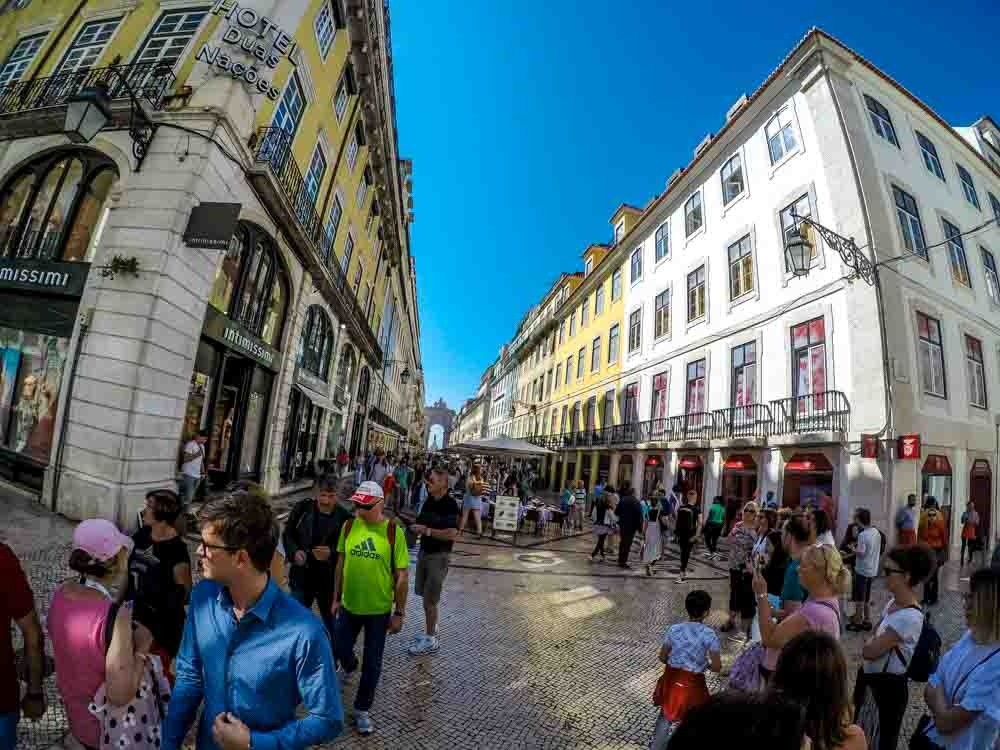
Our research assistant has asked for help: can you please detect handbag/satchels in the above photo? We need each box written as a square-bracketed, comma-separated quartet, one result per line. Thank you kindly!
[729, 643, 766, 695]
[87, 652, 162, 750]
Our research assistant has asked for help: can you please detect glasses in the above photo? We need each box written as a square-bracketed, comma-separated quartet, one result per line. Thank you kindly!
[198, 535, 242, 558]
[744, 509, 756, 513]
[882, 567, 907, 577]
[354, 497, 382, 510]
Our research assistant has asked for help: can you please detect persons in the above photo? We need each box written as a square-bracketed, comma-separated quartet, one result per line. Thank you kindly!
[588, 479, 1000, 750]
[336, 444, 587, 537]
[14, 375, 56, 463]
[182, 430, 207, 503]
[220, 413, 232, 472]
[1, 469, 458, 749]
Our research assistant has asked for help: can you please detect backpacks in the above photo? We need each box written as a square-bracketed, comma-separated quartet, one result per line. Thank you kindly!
[468, 476, 483, 496]
[676, 507, 697, 532]
[892, 604, 941, 683]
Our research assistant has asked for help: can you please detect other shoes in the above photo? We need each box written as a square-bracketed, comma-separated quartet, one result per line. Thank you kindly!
[862, 620, 873, 632]
[408, 633, 441, 655]
[619, 563, 631, 568]
[673, 578, 689, 585]
[719, 622, 736, 633]
[845, 623, 862, 632]
[646, 565, 652, 576]
[342, 670, 357, 684]
[352, 710, 373, 734]
[919, 598, 939, 606]
[457, 530, 465, 537]
[599, 558, 610, 563]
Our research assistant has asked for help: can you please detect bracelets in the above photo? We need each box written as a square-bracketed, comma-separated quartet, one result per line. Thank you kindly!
[757, 593, 768, 599]
[201, 473, 206, 474]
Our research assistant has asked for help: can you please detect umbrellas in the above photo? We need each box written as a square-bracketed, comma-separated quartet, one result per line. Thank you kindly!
[436, 436, 558, 459]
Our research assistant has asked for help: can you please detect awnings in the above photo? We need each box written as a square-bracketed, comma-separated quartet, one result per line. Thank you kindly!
[295, 382, 344, 418]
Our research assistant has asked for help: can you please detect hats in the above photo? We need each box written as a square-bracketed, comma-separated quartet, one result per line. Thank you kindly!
[71, 519, 131, 561]
[347, 480, 385, 505]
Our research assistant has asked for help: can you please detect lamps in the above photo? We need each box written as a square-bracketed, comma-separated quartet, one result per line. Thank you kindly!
[384, 357, 410, 385]
[783, 204, 877, 286]
[507, 400, 537, 418]
[65, 63, 162, 172]
